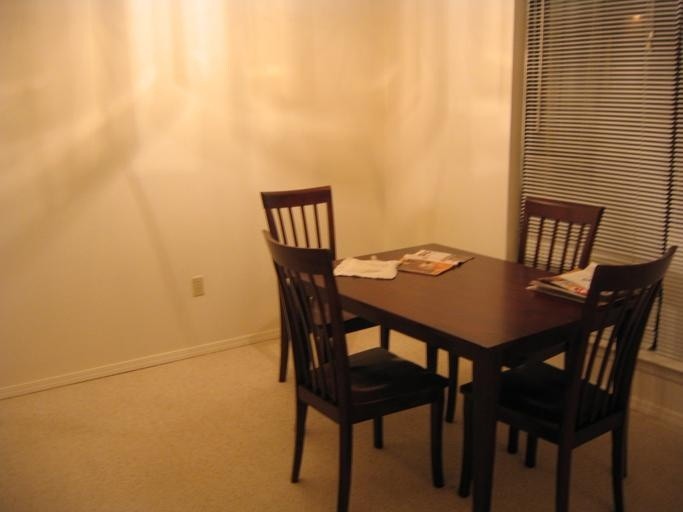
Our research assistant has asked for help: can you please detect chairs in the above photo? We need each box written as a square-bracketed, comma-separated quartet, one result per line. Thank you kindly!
[444, 196, 607, 453]
[260, 185, 390, 383]
[458, 245, 678, 511]
[262, 229, 452, 512]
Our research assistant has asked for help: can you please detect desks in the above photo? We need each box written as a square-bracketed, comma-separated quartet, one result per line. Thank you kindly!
[300, 242, 637, 512]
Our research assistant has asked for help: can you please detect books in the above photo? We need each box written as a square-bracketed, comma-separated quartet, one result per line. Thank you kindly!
[394, 249, 474, 276]
[526, 261, 614, 306]
